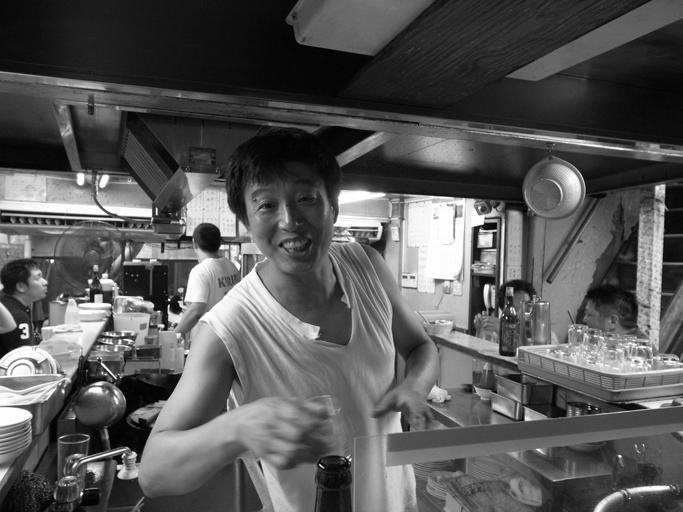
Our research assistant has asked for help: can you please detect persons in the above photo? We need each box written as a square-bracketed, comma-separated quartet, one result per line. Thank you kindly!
[135, 125, 443, 512]
[481, 278, 560, 377]
[0, 257, 48, 359]
[173, 222, 243, 350]
[581, 282, 662, 359]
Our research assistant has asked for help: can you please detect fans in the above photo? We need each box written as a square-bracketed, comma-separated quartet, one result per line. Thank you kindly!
[52, 220, 130, 296]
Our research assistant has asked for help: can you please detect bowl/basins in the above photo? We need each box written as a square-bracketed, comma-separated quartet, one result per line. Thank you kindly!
[565, 440, 607, 453]
[423, 319, 455, 338]
[522, 155, 586, 221]
[471, 382, 498, 403]
[92, 324, 138, 363]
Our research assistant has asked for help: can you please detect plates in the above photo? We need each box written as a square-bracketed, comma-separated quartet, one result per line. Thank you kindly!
[0, 405, 33, 465]
[77, 302, 112, 323]
[407, 415, 510, 502]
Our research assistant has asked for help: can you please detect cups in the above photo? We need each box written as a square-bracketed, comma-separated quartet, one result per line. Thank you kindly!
[550, 322, 679, 380]
[54, 431, 92, 494]
[301, 393, 354, 470]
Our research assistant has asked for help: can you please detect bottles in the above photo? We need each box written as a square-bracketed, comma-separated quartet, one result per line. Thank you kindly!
[497, 286, 518, 357]
[88, 263, 115, 306]
[471, 358, 496, 389]
[315, 454, 353, 512]
[64, 298, 80, 326]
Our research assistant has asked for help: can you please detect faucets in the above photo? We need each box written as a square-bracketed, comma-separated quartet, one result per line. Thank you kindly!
[70, 446, 139, 481]
[88, 357, 120, 384]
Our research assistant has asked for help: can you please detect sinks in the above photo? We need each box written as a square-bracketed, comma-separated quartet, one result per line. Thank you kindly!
[106, 458, 263, 512]
